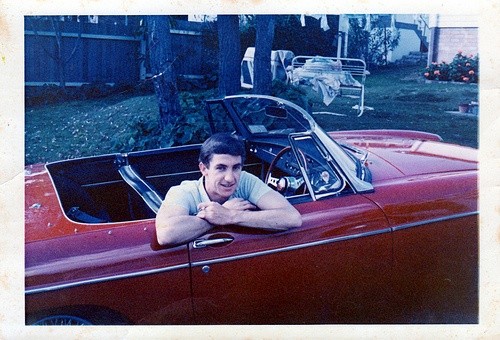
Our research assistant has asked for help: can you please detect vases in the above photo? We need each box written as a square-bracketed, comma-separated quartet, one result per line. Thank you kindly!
[459, 104, 468, 113]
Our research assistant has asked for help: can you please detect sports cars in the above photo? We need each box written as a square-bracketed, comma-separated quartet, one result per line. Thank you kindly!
[25, 91, 479, 323]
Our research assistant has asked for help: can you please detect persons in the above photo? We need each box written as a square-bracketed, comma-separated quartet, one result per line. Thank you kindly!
[153, 132, 304, 247]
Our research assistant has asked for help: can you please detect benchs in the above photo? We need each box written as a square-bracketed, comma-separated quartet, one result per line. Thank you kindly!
[287, 55, 368, 117]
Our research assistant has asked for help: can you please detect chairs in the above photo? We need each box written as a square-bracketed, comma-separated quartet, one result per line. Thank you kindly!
[113, 153, 164, 216]
